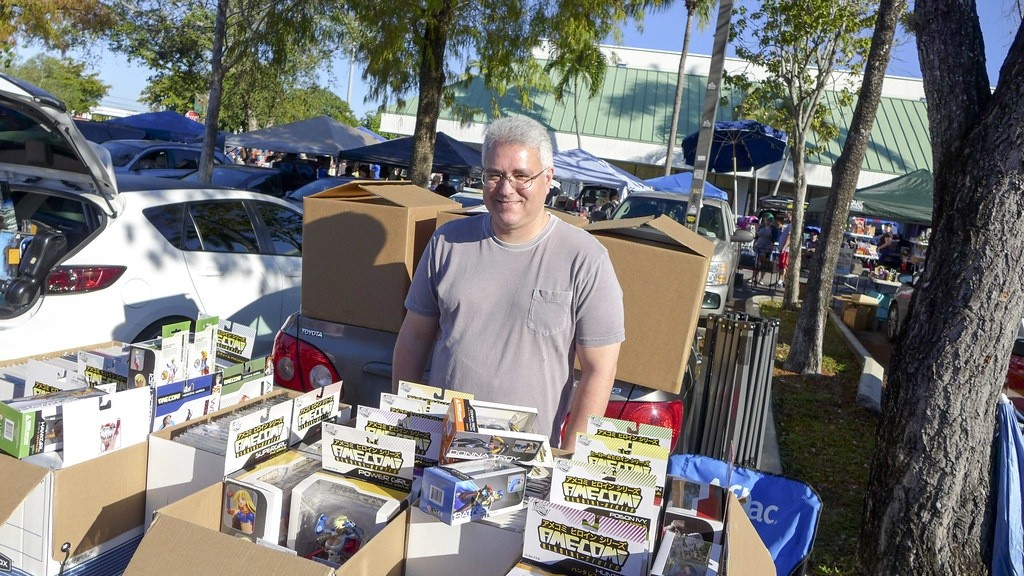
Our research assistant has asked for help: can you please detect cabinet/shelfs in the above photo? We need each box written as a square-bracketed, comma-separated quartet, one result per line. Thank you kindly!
[831, 232, 929, 318]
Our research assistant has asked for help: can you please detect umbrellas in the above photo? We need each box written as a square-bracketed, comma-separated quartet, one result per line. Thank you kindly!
[679, 120, 787, 223]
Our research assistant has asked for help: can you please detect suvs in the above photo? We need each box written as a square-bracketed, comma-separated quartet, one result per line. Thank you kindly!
[97, 138, 237, 184]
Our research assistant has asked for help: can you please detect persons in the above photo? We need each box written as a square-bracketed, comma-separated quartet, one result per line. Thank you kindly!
[389, 119, 627, 458]
[876, 221, 895, 266]
[806, 232, 820, 259]
[334, 160, 458, 201]
[743, 206, 796, 288]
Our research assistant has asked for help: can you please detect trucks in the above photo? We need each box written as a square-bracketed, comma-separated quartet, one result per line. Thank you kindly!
[572, 184, 621, 216]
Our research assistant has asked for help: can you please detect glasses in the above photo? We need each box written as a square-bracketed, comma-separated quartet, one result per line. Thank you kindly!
[479, 169, 548, 187]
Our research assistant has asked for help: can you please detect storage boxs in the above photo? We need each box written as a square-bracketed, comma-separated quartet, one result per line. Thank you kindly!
[121, 440, 422, 576]
[300, 180, 463, 333]
[573, 214, 717, 395]
[0, 340, 284, 576]
[503, 473, 778, 576]
[144, 380, 352, 534]
[434, 202, 589, 231]
[401, 446, 575, 576]
[831, 295, 882, 331]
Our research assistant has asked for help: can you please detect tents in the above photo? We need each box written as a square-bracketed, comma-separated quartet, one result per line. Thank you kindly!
[644, 171, 731, 203]
[331, 132, 487, 194]
[71, 105, 389, 176]
[550, 147, 650, 223]
[805, 169, 937, 238]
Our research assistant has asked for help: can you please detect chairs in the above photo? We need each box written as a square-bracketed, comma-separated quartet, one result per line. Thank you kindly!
[667, 454, 824, 576]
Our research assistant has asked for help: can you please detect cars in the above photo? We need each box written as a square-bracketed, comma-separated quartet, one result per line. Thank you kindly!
[887, 272, 1024, 357]
[175, 162, 290, 199]
[1, 70, 307, 380]
[287, 177, 355, 202]
[273, 303, 699, 456]
[601, 192, 757, 319]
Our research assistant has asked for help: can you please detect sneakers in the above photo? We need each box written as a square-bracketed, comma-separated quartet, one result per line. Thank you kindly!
[759, 278, 767, 285]
[747, 278, 752, 282]
[776, 279, 782, 286]
